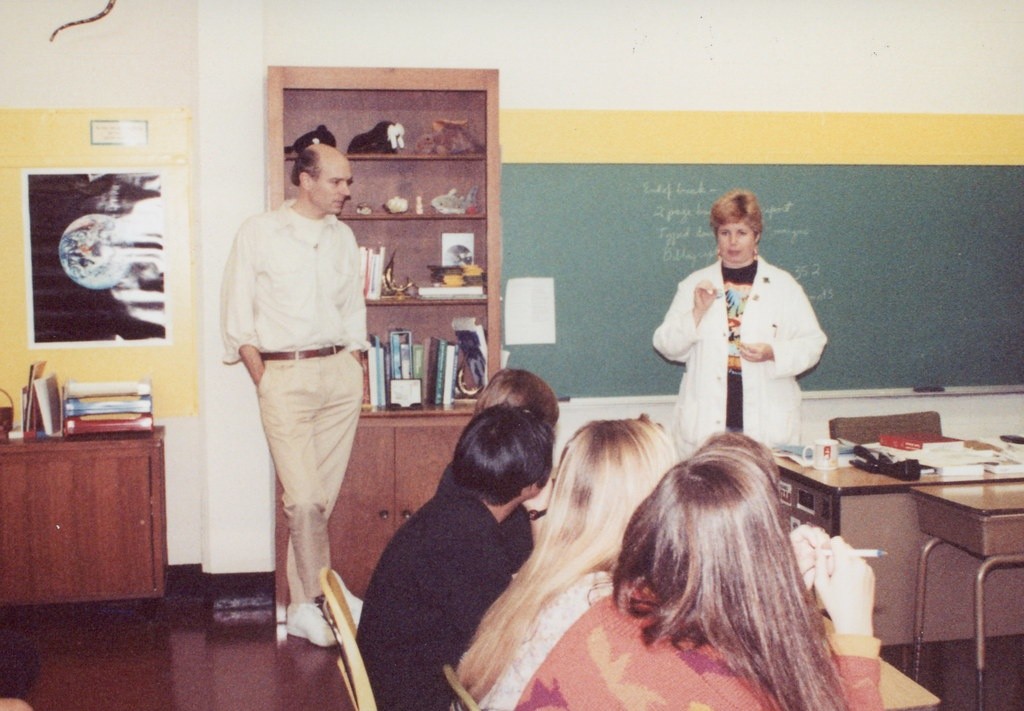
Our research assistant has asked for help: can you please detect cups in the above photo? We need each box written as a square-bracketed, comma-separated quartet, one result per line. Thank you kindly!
[801, 439, 839, 470]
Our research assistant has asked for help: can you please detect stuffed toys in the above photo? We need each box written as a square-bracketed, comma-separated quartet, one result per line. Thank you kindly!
[347, 120, 405, 154]
[415, 120, 481, 156]
[284, 124, 336, 154]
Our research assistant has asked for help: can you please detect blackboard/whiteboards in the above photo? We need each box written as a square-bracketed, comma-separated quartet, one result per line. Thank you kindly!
[498, 162, 1024, 406]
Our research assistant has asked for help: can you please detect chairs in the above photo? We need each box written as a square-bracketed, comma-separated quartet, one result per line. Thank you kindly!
[828, 411, 943, 443]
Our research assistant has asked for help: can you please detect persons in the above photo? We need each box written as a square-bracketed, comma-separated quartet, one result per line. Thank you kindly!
[358, 406, 556, 711]
[652, 192, 827, 462]
[450, 419, 678, 711]
[514, 433, 884, 711]
[220, 144, 365, 647]
[438, 369, 558, 573]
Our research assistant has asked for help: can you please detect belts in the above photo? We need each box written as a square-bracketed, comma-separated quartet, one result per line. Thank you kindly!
[261, 345, 345, 360]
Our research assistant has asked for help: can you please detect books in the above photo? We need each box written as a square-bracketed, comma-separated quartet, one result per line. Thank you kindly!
[20, 362, 149, 436]
[359, 246, 386, 299]
[362, 320, 487, 411]
[775, 433, 1024, 478]
[413, 283, 486, 299]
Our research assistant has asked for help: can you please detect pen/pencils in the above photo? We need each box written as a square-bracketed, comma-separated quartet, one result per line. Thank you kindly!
[812, 547, 891, 560]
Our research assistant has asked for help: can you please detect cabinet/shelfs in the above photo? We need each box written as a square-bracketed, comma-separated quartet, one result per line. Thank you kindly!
[1, 425, 170, 606]
[266, 62, 501, 609]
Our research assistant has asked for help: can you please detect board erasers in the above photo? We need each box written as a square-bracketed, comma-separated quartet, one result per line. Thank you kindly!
[912, 384, 946, 392]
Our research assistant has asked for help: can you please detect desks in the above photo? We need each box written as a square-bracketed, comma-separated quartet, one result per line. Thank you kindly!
[769, 442, 1024, 647]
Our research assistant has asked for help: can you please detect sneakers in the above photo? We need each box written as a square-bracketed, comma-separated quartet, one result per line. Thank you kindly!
[286, 603, 336, 647]
